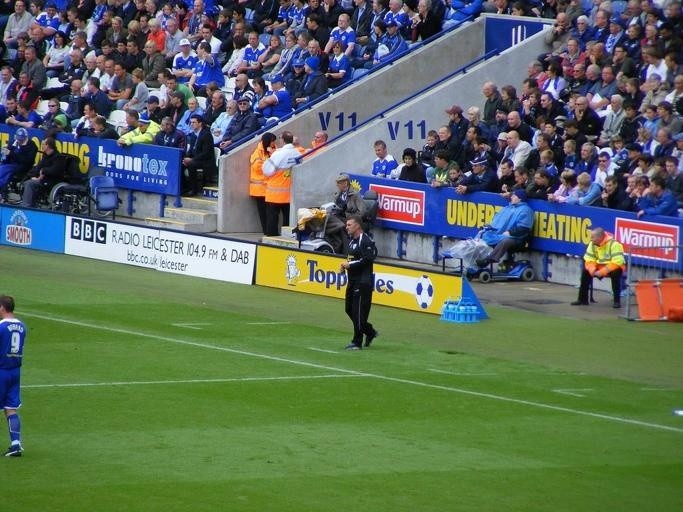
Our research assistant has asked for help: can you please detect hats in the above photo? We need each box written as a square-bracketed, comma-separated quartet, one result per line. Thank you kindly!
[178, 37, 192, 47]
[470, 155, 488, 167]
[497, 132, 509, 142]
[237, 57, 322, 103]
[445, 105, 464, 114]
[335, 173, 349, 183]
[143, 95, 160, 106]
[384, 19, 399, 26]
[513, 188, 525, 201]
[609, 16, 626, 27]
[138, 110, 151, 125]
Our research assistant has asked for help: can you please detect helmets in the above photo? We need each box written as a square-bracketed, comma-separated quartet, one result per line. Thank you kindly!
[12, 128, 30, 141]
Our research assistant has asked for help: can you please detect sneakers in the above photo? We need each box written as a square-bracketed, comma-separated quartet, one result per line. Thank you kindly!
[364, 330, 378, 347]
[570, 298, 590, 306]
[345, 343, 363, 351]
[3, 443, 23, 457]
[612, 301, 622, 310]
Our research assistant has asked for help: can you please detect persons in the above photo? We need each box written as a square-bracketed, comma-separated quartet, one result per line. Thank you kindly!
[0, 296, 27, 457]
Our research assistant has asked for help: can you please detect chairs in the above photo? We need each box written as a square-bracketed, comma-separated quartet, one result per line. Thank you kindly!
[87, 176, 120, 221]
[292, 34, 421, 98]
[30, 35, 166, 144]
[154, 34, 295, 179]
[588, 254, 628, 304]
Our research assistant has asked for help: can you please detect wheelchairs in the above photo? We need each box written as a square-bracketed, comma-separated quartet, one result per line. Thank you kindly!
[300, 190, 379, 258]
[2, 172, 112, 217]
[461, 228, 537, 283]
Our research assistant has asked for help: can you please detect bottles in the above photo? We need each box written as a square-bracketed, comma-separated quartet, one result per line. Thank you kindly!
[671, 409, 683, 415]
